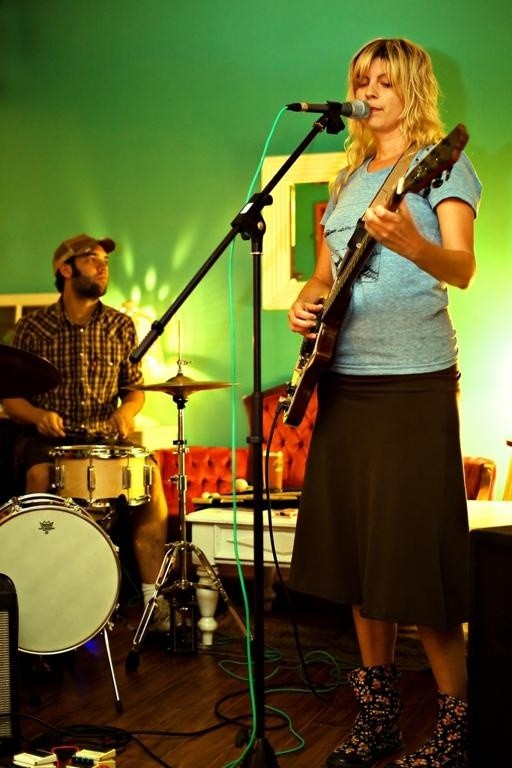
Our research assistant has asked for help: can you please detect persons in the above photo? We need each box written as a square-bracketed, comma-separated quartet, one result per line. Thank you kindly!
[288, 34, 481, 766]
[1, 233, 191, 645]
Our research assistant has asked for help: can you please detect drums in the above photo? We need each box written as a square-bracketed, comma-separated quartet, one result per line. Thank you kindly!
[0, 492, 120, 655]
[47, 443, 152, 507]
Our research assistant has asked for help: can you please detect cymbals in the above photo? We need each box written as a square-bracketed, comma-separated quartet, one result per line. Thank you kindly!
[120, 374, 237, 397]
[0, 343, 62, 399]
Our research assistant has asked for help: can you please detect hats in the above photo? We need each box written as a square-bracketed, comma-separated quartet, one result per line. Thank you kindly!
[52, 235, 115, 276]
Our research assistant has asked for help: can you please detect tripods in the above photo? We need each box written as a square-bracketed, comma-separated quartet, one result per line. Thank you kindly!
[126, 409, 252, 678]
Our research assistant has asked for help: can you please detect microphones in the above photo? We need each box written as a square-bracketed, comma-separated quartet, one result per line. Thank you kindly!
[283, 97, 370, 120]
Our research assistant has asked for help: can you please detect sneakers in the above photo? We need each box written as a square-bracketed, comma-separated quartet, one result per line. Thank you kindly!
[144, 593, 195, 635]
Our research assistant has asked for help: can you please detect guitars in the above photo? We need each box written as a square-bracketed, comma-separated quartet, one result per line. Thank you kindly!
[284, 123, 469, 427]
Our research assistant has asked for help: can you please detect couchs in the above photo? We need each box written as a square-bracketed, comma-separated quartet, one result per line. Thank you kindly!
[145, 381, 496, 526]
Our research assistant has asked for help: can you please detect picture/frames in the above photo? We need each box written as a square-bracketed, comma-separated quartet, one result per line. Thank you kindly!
[259, 152, 365, 311]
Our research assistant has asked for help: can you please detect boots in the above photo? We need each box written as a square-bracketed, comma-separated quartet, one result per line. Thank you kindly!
[324, 663, 401, 768]
[387, 691, 472, 766]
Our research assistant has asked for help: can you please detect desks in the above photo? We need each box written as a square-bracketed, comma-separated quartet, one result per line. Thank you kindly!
[184, 499, 512, 649]
